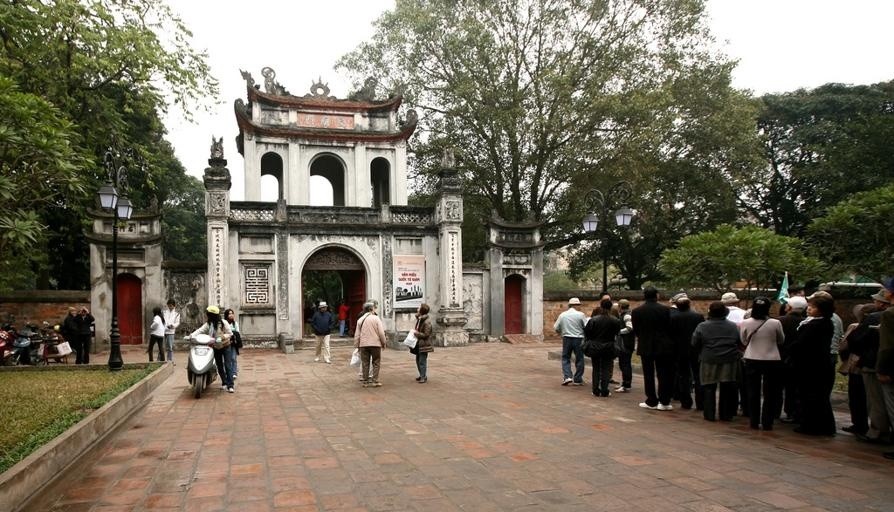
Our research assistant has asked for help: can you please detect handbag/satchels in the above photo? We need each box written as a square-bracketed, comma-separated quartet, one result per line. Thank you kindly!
[351, 348, 362, 365]
[57, 341, 73, 355]
[403, 330, 419, 349]
[410, 347, 418, 354]
[838, 354, 860, 374]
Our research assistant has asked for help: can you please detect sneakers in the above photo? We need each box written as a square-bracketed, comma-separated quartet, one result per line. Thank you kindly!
[325, 359, 332, 364]
[561, 377, 572, 385]
[314, 358, 322, 361]
[358, 371, 372, 381]
[640, 402, 656, 410]
[371, 380, 383, 387]
[657, 402, 673, 411]
[615, 384, 632, 389]
[614, 386, 631, 393]
[591, 391, 612, 397]
[574, 382, 584, 386]
[678, 403, 894, 444]
[364, 380, 370, 386]
[221, 371, 237, 393]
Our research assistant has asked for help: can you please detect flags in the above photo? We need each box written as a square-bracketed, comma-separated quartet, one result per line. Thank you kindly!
[776, 271, 790, 306]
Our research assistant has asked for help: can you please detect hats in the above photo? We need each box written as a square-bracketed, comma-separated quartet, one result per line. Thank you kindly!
[205, 305, 220, 315]
[879, 277, 893, 291]
[719, 293, 740, 304]
[783, 295, 808, 310]
[319, 302, 328, 308]
[567, 298, 581, 305]
[871, 289, 891, 305]
[670, 294, 688, 303]
[806, 291, 832, 301]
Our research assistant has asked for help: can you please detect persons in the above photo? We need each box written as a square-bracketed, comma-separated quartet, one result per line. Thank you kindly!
[305, 299, 352, 338]
[184, 306, 234, 393]
[691, 290, 893, 459]
[222, 309, 239, 380]
[161, 300, 181, 366]
[311, 300, 335, 363]
[415, 304, 434, 383]
[353, 302, 387, 388]
[148, 307, 167, 361]
[355, 298, 379, 381]
[78, 307, 95, 364]
[554, 286, 706, 411]
[63, 306, 83, 365]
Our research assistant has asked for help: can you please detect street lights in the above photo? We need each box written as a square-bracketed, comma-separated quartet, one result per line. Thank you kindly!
[99, 147, 135, 373]
[581, 180, 634, 295]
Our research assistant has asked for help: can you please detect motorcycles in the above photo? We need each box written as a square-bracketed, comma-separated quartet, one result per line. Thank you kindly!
[184, 334, 218, 399]
[0, 315, 67, 366]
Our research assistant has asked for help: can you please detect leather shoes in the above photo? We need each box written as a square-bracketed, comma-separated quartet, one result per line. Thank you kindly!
[609, 379, 620, 384]
[416, 376, 428, 383]
